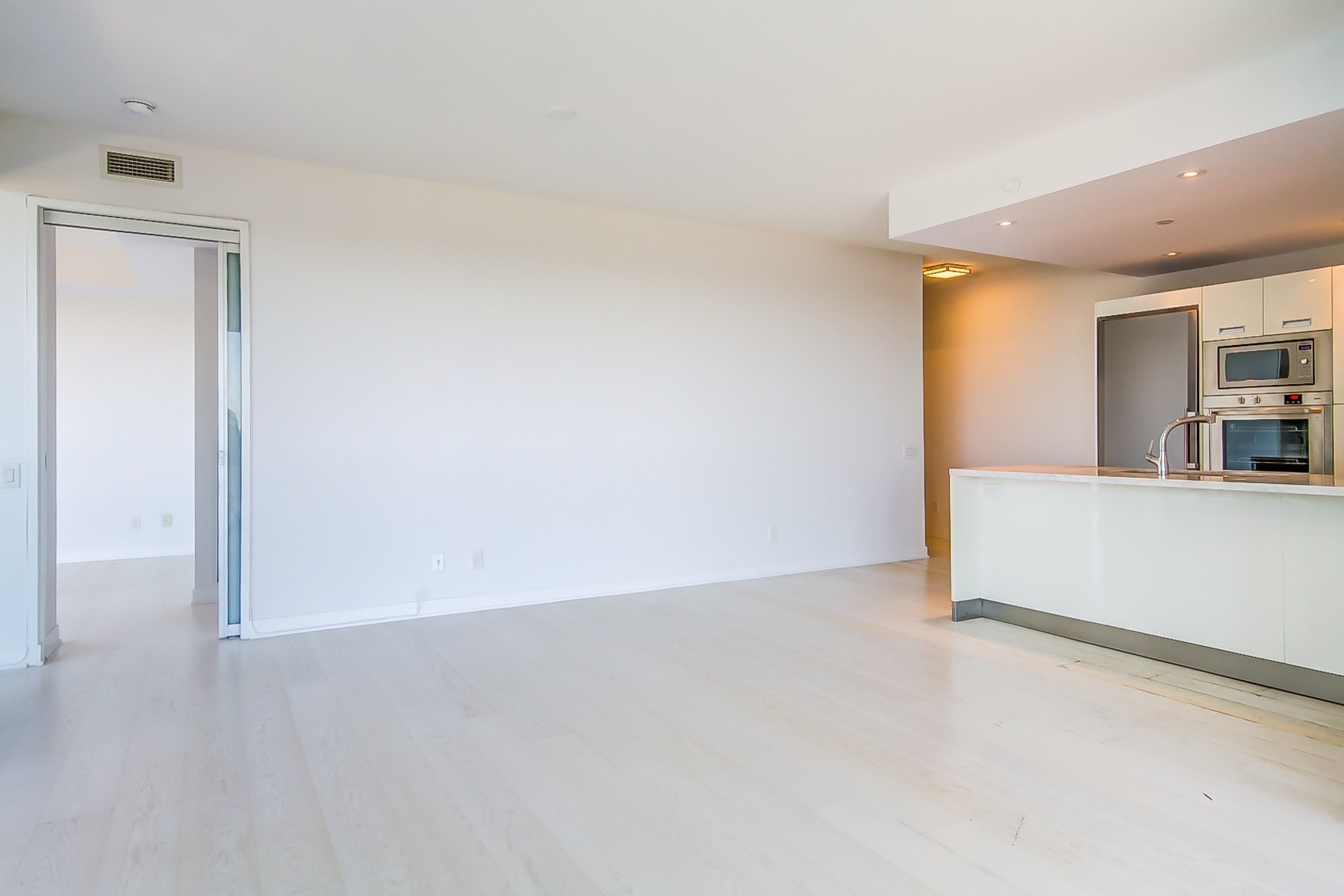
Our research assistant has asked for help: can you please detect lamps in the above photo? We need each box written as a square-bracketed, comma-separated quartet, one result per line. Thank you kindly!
[923, 263, 975, 281]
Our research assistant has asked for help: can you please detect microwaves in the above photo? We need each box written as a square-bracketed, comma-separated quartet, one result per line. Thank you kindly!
[1203, 329, 1333, 396]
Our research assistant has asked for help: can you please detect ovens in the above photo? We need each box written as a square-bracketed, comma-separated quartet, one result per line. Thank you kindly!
[1204, 392, 1334, 476]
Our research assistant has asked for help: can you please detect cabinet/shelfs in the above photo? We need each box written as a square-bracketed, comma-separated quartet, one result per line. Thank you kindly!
[1202, 269, 1332, 342]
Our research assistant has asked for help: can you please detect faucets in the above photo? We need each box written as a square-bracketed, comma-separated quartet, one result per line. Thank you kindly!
[1142, 414, 1215, 475]
